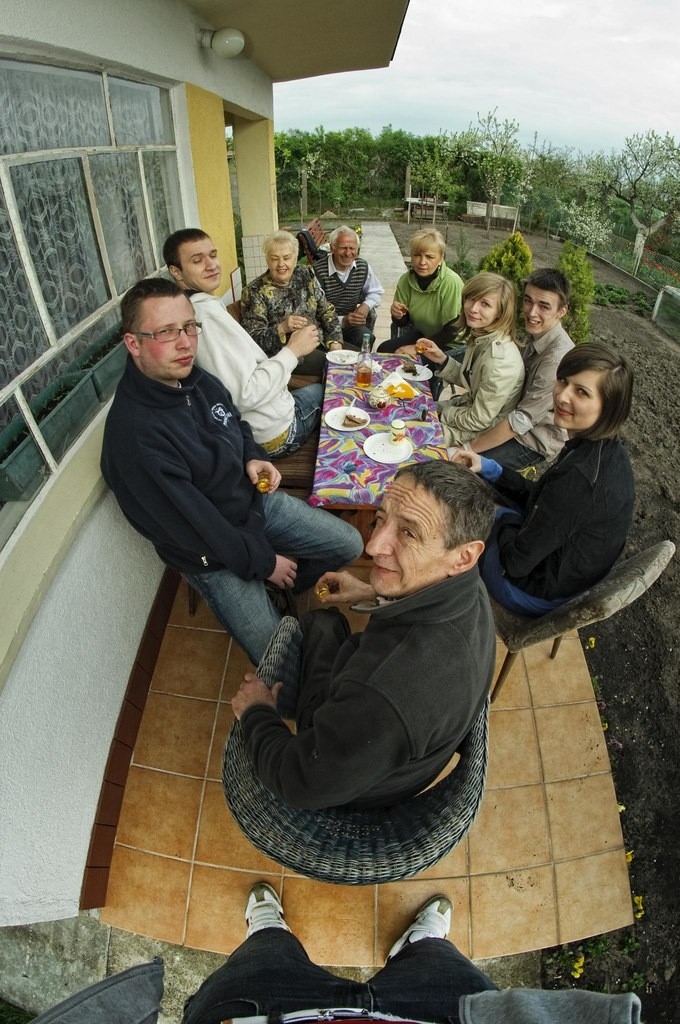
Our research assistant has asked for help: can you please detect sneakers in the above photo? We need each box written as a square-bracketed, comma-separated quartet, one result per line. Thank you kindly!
[385, 894, 452, 965]
[245, 882, 292, 941]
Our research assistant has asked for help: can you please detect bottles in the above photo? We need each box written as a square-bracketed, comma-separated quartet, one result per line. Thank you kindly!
[368, 385, 391, 410]
[355, 334, 372, 390]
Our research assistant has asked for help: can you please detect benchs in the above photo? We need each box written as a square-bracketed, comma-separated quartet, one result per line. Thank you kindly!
[299, 218, 333, 265]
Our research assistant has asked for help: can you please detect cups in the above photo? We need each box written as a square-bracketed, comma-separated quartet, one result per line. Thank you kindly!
[399, 302, 408, 315]
[389, 419, 406, 443]
[256, 472, 271, 494]
[415, 339, 428, 354]
[310, 583, 332, 600]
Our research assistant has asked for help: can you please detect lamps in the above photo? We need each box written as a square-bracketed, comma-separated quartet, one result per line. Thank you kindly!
[197, 27, 245, 58]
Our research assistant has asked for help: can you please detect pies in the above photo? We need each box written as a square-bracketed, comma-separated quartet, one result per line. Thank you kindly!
[390, 382, 414, 398]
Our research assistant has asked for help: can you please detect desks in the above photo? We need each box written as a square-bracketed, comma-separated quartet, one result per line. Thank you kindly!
[309, 352, 450, 566]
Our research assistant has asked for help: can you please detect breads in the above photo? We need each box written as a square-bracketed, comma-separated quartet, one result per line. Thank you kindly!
[314, 583, 330, 600]
[415, 342, 426, 353]
[404, 362, 416, 373]
[256, 472, 271, 494]
[344, 415, 368, 427]
[400, 305, 407, 314]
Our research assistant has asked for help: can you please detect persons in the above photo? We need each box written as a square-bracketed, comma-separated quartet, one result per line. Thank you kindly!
[241, 230, 362, 376]
[415, 272, 525, 449]
[451, 342, 636, 619]
[376, 227, 465, 381]
[232, 459, 496, 812]
[448, 268, 577, 473]
[25, 882, 641, 1024]
[162, 228, 325, 459]
[99, 279, 364, 668]
[311, 226, 386, 354]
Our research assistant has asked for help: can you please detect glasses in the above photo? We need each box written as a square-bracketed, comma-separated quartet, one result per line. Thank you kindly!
[140, 322, 204, 343]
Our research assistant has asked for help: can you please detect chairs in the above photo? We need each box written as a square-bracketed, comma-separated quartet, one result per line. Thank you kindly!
[187, 291, 537, 617]
[222, 617, 490, 886]
[489, 538, 677, 707]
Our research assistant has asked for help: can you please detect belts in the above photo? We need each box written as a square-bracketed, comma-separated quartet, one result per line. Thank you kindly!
[267, 414, 297, 460]
[221, 1008, 433, 1024]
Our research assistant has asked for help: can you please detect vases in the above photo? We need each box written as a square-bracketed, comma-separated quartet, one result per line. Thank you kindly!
[0, 321, 128, 503]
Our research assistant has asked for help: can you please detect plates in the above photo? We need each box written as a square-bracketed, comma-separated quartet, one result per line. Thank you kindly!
[324, 406, 371, 432]
[369, 372, 421, 397]
[325, 349, 359, 365]
[362, 433, 413, 464]
[395, 363, 433, 382]
